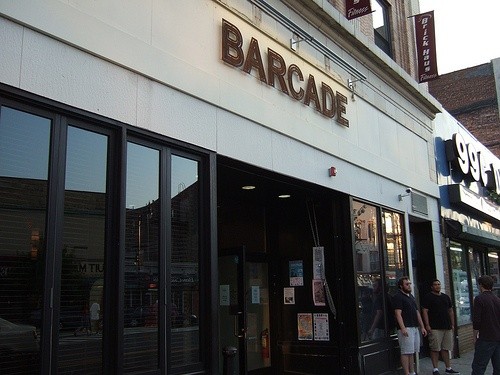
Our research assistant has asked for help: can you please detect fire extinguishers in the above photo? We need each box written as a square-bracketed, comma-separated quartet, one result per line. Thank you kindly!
[262, 328, 269, 358]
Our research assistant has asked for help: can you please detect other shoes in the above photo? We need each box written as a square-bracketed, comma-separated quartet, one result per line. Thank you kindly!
[445, 369, 460, 375]
[433, 371, 440, 375]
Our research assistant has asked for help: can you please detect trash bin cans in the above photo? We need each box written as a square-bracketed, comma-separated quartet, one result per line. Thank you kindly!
[223, 346, 238, 375]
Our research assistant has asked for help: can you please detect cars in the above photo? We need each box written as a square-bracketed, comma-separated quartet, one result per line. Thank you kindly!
[0, 318, 40, 364]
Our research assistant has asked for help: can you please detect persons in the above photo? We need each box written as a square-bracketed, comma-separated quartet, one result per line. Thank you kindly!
[368, 277, 394, 334]
[471, 275, 500, 375]
[424, 280, 460, 375]
[89, 298, 100, 336]
[391, 276, 428, 375]
